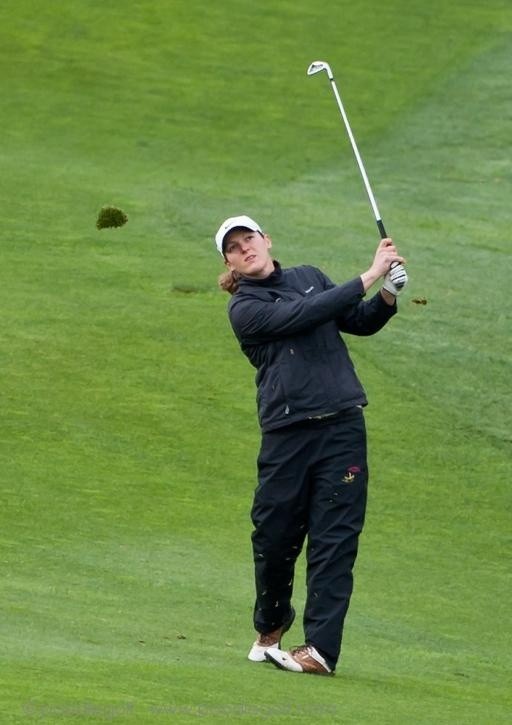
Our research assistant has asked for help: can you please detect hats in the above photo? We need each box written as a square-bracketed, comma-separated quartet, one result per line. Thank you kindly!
[215, 215, 265, 261]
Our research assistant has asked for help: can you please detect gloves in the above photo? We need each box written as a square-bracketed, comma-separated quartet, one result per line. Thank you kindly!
[382, 261, 408, 297]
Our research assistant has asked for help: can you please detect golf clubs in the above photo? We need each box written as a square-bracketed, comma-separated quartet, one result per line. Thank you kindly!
[307, 61, 405, 289]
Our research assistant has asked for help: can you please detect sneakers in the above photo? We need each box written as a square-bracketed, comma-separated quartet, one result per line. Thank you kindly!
[248, 604, 296, 662]
[263, 643, 336, 677]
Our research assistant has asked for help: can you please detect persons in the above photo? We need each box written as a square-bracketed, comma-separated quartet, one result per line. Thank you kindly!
[211, 215, 409, 674]
